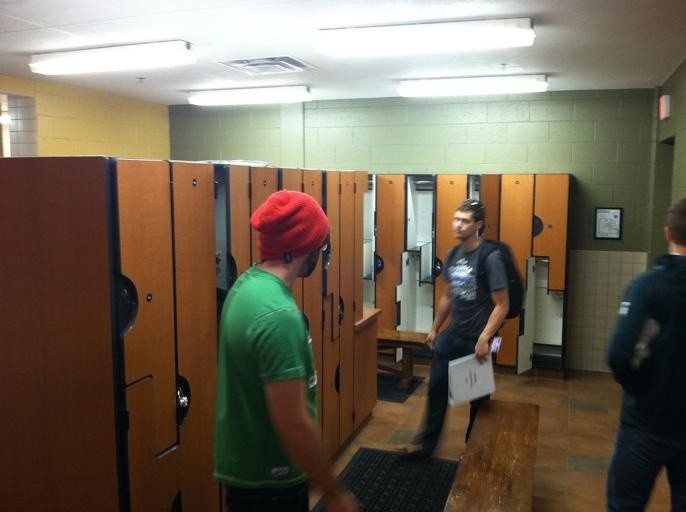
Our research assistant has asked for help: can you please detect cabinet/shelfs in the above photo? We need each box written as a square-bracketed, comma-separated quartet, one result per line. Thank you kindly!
[363, 168, 571, 399]
[1, 157, 382, 512]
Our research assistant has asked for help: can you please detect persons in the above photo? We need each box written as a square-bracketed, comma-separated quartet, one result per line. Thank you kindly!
[397, 199, 526, 461]
[209, 188, 363, 510]
[603, 200, 686, 512]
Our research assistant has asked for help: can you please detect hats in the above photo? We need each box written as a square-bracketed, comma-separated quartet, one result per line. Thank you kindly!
[250, 190, 329, 260]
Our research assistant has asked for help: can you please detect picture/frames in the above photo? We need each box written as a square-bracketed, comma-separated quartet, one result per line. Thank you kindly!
[594, 206, 623, 241]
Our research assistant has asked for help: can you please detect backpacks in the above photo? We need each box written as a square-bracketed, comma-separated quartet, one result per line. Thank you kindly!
[445, 233, 523, 319]
